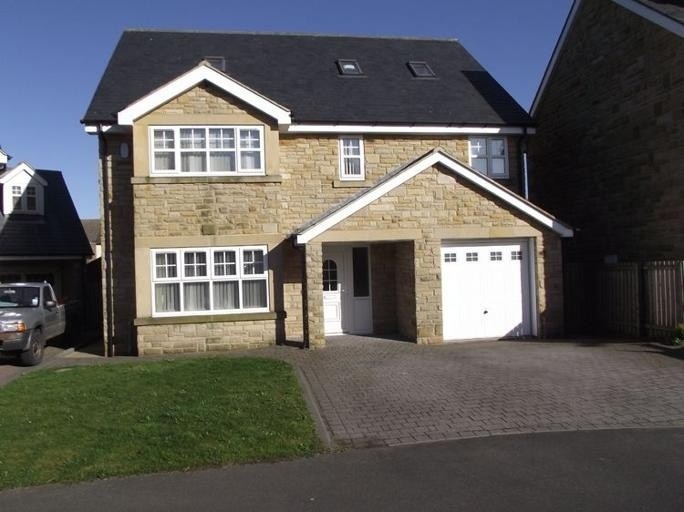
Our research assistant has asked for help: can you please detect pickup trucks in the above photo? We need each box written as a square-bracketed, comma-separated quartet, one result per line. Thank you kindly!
[1, 280, 85, 368]
